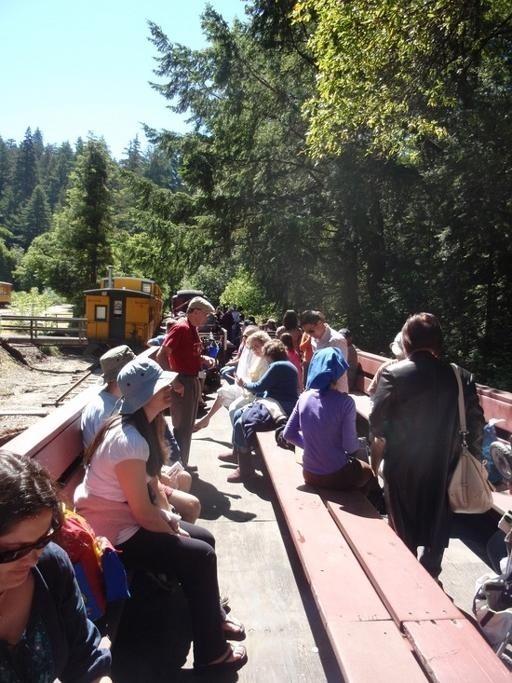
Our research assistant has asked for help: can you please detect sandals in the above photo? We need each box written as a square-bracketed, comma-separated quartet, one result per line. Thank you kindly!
[192, 423, 208, 432]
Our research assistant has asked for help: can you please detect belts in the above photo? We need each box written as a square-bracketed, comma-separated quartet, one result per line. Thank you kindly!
[181, 373, 199, 378]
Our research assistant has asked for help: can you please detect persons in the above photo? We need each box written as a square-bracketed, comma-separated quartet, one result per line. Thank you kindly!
[73, 358, 247, 672]
[156, 296, 216, 480]
[0, 448, 114, 683]
[80, 344, 201, 524]
[147, 319, 207, 418]
[193, 305, 405, 516]
[368, 313, 485, 581]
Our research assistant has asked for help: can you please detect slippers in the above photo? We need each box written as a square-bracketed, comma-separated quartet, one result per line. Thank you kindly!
[220, 615, 246, 638]
[193, 645, 247, 669]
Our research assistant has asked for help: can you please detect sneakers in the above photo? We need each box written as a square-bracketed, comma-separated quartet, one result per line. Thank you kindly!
[227, 467, 254, 483]
[218, 454, 238, 464]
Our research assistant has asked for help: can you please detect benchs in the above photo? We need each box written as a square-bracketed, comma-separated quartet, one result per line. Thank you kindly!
[255, 431, 512, 683]
[1, 345, 163, 578]
[348, 342, 512, 512]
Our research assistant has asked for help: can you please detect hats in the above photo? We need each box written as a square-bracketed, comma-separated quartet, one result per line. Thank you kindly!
[115, 357, 179, 414]
[97, 344, 138, 385]
[188, 296, 216, 313]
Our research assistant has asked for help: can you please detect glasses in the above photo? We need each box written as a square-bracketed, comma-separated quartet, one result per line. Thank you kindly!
[0, 516, 61, 564]
[306, 324, 317, 334]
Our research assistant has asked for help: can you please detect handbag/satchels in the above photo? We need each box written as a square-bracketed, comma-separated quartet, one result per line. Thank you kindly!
[55, 501, 131, 622]
[448, 440, 496, 515]
